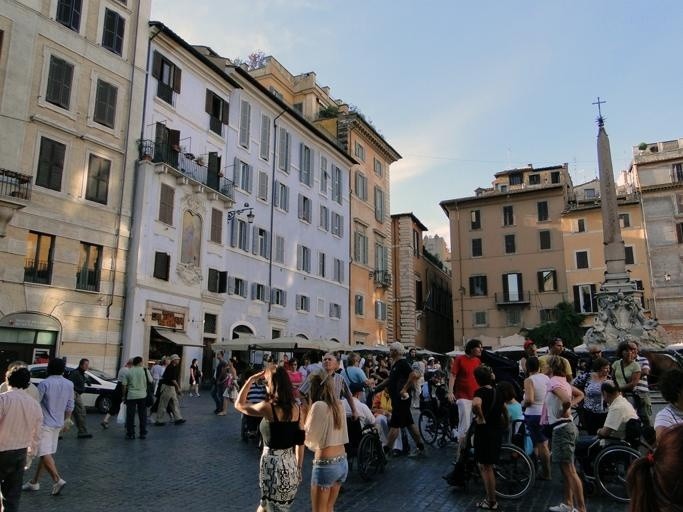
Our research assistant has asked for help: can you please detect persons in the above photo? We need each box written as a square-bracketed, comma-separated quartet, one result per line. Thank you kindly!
[519, 356, 552, 481]
[375, 353, 391, 385]
[285, 357, 305, 399]
[340, 352, 375, 395]
[653, 368, 683, 445]
[405, 348, 422, 366]
[100, 358, 134, 429]
[0, 364, 43, 511]
[242, 369, 267, 405]
[424, 356, 441, 372]
[518, 339, 539, 378]
[151, 360, 161, 396]
[628, 340, 652, 426]
[573, 379, 644, 487]
[0, 360, 39, 405]
[145, 355, 176, 423]
[441, 381, 533, 487]
[234, 363, 305, 512]
[571, 357, 613, 435]
[372, 386, 404, 455]
[446, 339, 482, 461]
[537, 353, 587, 512]
[210, 349, 229, 414]
[419, 370, 458, 441]
[215, 358, 239, 416]
[536, 337, 573, 384]
[188, 357, 202, 398]
[470, 366, 502, 509]
[410, 354, 424, 408]
[371, 342, 427, 456]
[117, 356, 153, 440]
[153, 354, 186, 426]
[625, 423, 682, 512]
[341, 382, 389, 457]
[296, 352, 359, 494]
[586, 345, 612, 377]
[19, 357, 75, 497]
[300, 369, 350, 512]
[610, 339, 642, 414]
[56, 359, 94, 439]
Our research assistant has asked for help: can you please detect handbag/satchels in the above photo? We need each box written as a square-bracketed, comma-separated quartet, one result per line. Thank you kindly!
[197, 370, 201, 377]
[543, 420, 572, 438]
[633, 390, 645, 408]
[144, 368, 154, 407]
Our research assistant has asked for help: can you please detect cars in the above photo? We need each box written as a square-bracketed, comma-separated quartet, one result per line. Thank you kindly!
[25, 363, 120, 414]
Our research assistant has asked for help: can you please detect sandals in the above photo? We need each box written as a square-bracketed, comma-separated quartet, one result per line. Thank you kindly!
[477, 498, 499, 506]
[475, 501, 498, 510]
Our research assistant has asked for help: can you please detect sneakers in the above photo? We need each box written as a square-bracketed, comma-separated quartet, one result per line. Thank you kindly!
[195, 393, 200, 397]
[77, 433, 92, 439]
[125, 434, 135, 438]
[189, 392, 193, 397]
[21, 479, 40, 491]
[408, 448, 427, 457]
[155, 421, 166, 426]
[170, 417, 175, 423]
[101, 422, 109, 429]
[138, 436, 146, 439]
[51, 479, 66, 496]
[441, 471, 455, 480]
[549, 503, 578, 512]
[175, 419, 186, 425]
[124, 423, 136, 427]
[392, 449, 401, 456]
[146, 416, 155, 424]
[446, 478, 464, 485]
[217, 411, 227, 416]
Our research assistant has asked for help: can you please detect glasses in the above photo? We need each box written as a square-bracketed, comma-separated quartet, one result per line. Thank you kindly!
[591, 350, 602, 354]
[356, 359, 361, 362]
[291, 364, 297, 366]
[217, 354, 220, 355]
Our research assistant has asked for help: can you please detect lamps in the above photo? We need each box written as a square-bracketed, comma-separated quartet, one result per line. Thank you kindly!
[226, 206, 256, 225]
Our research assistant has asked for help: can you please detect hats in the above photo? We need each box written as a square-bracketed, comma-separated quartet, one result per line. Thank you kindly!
[524, 340, 536, 349]
[349, 382, 366, 393]
[170, 354, 180, 361]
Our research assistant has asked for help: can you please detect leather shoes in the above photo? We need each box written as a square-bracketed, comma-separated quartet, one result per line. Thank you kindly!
[215, 407, 223, 414]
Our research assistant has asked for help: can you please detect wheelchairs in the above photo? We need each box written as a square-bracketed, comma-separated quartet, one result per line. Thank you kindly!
[239, 411, 264, 447]
[583, 418, 656, 503]
[417, 392, 459, 448]
[441, 419, 533, 499]
[344, 417, 387, 479]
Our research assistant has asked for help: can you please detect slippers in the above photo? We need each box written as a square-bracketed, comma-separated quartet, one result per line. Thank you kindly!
[535, 472, 552, 480]
